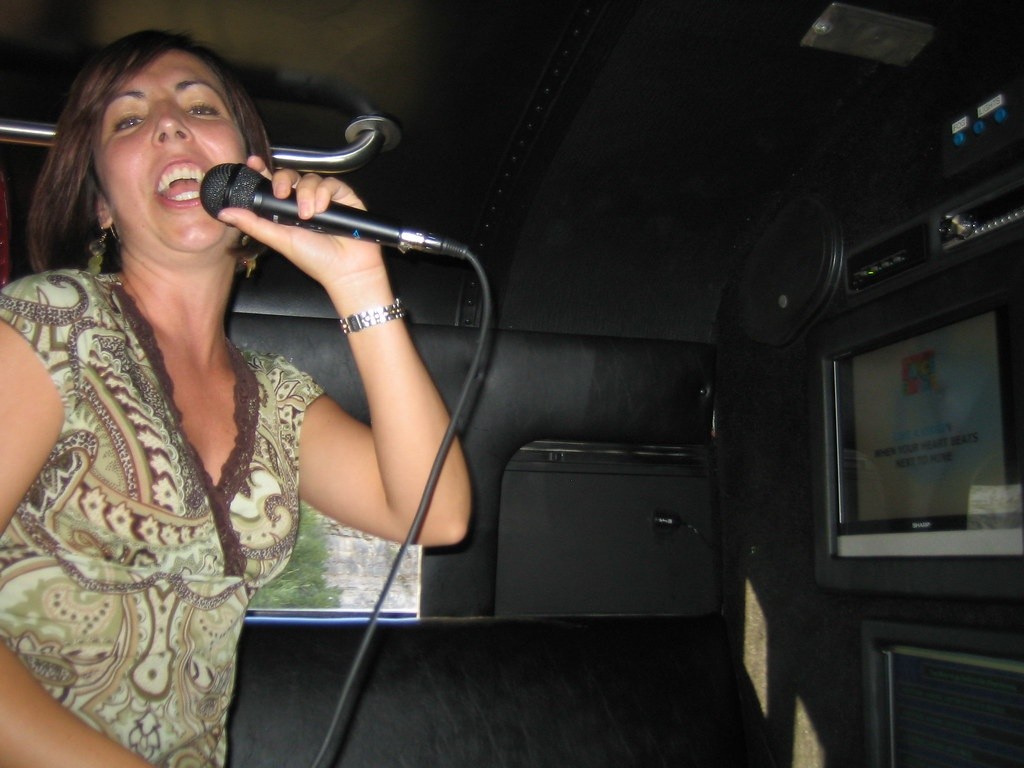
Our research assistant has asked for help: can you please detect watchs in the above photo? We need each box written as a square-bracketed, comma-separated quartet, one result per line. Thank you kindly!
[340, 296, 408, 337]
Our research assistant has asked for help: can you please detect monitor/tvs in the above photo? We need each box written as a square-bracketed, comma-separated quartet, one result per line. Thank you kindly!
[803, 247, 1023, 599]
[856, 619, 1024, 768]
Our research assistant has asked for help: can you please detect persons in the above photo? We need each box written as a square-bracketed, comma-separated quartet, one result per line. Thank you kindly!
[1, 30, 475, 768]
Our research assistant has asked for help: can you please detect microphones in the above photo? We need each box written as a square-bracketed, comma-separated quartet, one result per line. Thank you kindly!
[197, 163, 470, 258]
